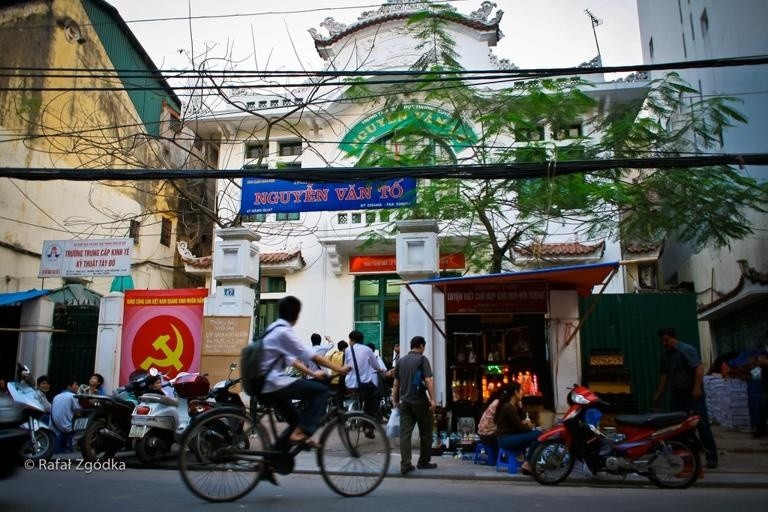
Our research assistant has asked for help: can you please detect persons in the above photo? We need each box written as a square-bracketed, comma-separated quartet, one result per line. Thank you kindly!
[144, 375, 165, 395]
[76, 373, 105, 396]
[476, 383, 507, 447]
[48, 378, 85, 437]
[303, 333, 399, 439]
[493, 381, 544, 476]
[0, 379, 8, 393]
[35, 376, 51, 424]
[651, 327, 718, 470]
[391, 337, 438, 475]
[256, 295, 353, 486]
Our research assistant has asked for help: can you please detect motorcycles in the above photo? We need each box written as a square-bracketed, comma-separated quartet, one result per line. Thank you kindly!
[8, 358, 254, 479]
[530, 382, 715, 490]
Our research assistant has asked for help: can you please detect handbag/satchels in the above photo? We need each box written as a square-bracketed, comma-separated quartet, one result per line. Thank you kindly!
[412, 369, 429, 391]
[359, 381, 382, 405]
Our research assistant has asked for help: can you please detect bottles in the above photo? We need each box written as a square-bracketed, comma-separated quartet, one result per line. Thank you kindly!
[451, 367, 538, 402]
[431, 429, 481, 449]
[457, 449, 463, 465]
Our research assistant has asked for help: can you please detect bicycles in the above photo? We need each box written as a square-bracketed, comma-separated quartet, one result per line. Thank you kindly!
[176, 364, 393, 505]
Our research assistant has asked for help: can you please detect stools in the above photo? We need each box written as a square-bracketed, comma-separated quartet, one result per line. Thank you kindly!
[55, 434, 74, 453]
[473, 441, 528, 474]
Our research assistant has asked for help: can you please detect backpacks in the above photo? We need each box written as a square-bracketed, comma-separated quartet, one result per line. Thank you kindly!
[240, 324, 291, 397]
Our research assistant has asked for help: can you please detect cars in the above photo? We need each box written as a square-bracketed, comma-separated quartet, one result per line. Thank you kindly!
[0, 389, 27, 479]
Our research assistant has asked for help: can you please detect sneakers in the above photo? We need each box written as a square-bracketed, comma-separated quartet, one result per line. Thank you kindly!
[364, 428, 376, 439]
[402, 465, 414, 475]
[418, 462, 437, 468]
[705, 460, 717, 468]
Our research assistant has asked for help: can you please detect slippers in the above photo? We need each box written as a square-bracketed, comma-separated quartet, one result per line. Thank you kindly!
[290, 435, 322, 451]
